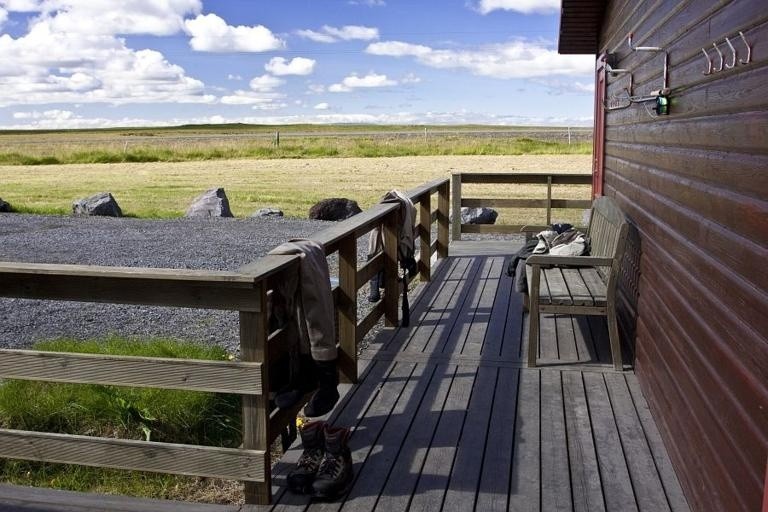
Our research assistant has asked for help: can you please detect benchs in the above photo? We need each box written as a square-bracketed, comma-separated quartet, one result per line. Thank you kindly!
[521, 193, 630, 372]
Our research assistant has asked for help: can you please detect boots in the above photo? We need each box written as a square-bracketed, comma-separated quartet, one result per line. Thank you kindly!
[287, 421, 327, 495]
[312, 425, 353, 498]
